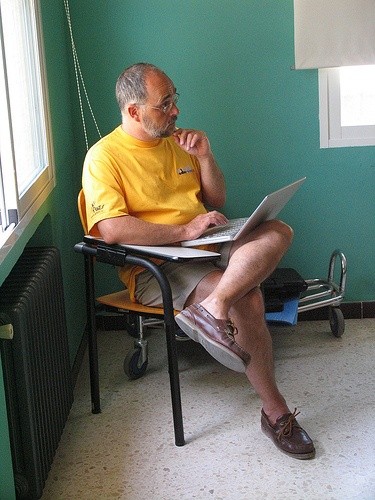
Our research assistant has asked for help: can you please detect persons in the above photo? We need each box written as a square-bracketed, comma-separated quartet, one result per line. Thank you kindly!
[81, 63, 316, 460]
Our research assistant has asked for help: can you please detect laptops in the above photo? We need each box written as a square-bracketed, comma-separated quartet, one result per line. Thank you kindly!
[181, 176, 307, 247]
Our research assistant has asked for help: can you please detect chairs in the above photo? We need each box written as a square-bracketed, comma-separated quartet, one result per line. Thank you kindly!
[74, 189, 221, 447]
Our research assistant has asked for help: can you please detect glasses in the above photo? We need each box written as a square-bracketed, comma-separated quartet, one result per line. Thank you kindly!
[134, 93, 180, 113]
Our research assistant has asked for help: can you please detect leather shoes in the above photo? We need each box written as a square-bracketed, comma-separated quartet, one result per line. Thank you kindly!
[175, 302, 251, 373]
[261, 408, 315, 460]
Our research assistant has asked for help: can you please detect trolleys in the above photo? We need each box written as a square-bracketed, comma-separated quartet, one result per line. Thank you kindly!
[121, 245, 348, 381]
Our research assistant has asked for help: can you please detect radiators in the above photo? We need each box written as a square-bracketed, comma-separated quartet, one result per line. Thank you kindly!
[0, 246, 74, 500]
